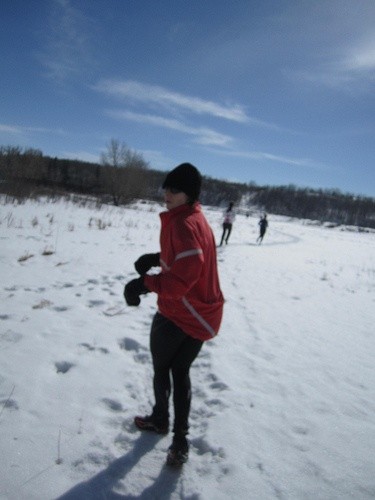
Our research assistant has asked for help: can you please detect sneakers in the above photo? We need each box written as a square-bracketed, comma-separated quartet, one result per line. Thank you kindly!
[135, 416, 169, 435]
[173, 437, 188, 463]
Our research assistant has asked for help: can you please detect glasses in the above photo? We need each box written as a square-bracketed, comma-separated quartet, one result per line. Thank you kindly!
[165, 186, 182, 193]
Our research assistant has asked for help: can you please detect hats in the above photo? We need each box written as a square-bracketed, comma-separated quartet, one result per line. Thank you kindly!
[162, 163, 202, 201]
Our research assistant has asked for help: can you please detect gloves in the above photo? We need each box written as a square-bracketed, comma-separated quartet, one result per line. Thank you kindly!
[124, 276, 147, 306]
[135, 254, 160, 275]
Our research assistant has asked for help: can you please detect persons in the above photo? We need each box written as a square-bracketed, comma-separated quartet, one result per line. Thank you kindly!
[220, 202, 236, 245]
[123, 163, 225, 465]
[257, 213, 269, 244]
